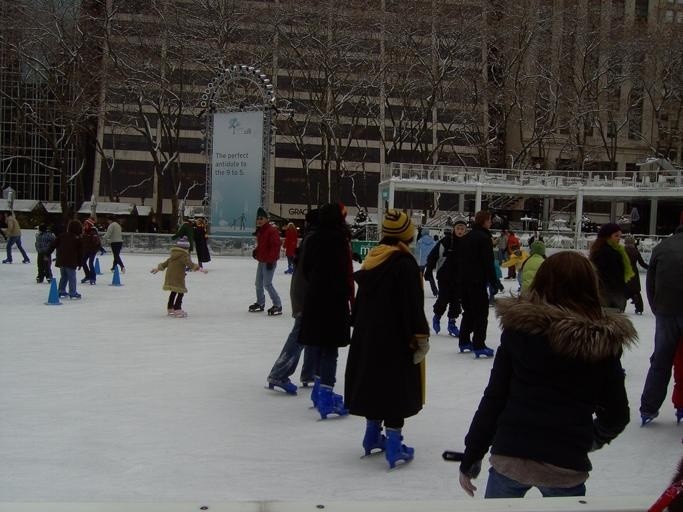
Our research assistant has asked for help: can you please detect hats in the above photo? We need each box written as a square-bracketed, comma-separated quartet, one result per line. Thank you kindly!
[453, 215, 467, 228]
[257, 207, 267, 217]
[176, 236, 190, 248]
[338, 202, 347, 219]
[531, 241, 545, 256]
[624, 235, 635, 244]
[84, 217, 96, 226]
[382, 209, 416, 242]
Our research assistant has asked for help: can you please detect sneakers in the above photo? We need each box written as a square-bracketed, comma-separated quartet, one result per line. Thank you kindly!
[641, 409, 659, 418]
[269, 375, 413, 469]
[433, 316, 494, 357]
[249, 302, 265, 309]
[169, 308, 187, 315]
[285, 268, 292, 273]
[2, 257, 96, 298]
[268, 306, 282, 312]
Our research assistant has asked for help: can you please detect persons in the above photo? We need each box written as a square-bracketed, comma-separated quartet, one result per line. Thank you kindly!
[344, 223, 356, 311]
[417, 220, 546, 307]
[424, 220, 466, 338]
[171, 216, 211, 275]
[35, 213, 128, 300]
[150, 236, 208, 319]
[247, 208, 356, 420]
[0, 211, 31, 264]
[647, 335, 681, 423]
[637, 222, 683, 425]
[588, 222, 648, 314]
[451, 209, 504, 360]
[650, 456, 683, 512]
[344, 210, 431, 469]
[442, 250, 640, 499]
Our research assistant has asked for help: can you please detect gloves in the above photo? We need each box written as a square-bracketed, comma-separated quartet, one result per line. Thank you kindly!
[413, 339, 429, 365]
[253, 249, 257, 259]
[196, 267, 204, 272]
[150, 268, 160, 275]
[424, 266, 433, 282]
[266, 263, 273, 270]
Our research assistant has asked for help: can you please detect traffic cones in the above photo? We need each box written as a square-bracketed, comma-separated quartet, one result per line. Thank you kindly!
[94, 257, 102, 276]
[108, 265, 124, 287]
[44, 277, 64, 306]
